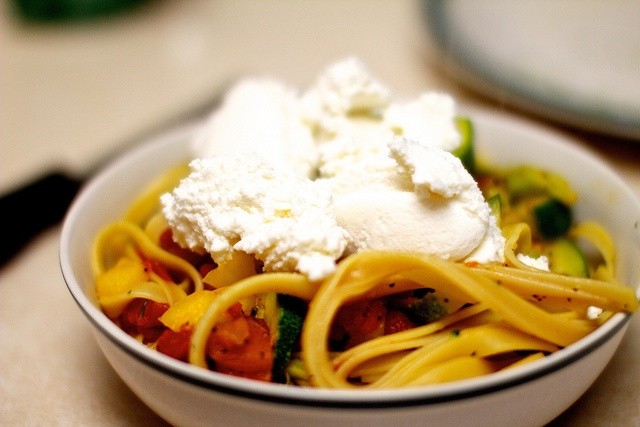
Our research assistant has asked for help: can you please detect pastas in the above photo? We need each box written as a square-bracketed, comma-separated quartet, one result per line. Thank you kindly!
[89, 141, 636, 392]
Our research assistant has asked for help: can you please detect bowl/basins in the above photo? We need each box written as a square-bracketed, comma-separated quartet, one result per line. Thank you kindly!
[58, 95, 636, 427]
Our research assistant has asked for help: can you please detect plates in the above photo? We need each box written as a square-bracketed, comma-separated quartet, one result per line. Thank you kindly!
[401, 0, 635, 161]
